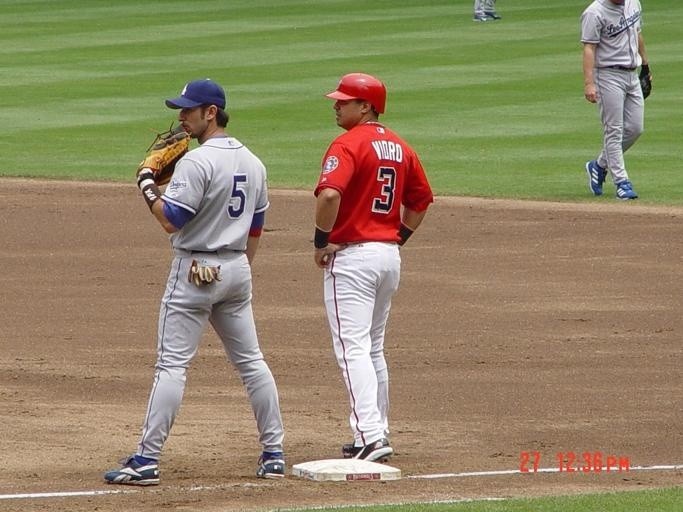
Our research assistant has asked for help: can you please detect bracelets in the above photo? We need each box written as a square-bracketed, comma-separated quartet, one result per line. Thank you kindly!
[140, 183, 161, 216]
[396, 222, 414, 246]
[312, 224, 331, 249]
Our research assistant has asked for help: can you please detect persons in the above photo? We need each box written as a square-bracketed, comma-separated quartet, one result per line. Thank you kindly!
[473, 1, 501, 23]
[578, 0, 652, 201]
[309, 73, 434, 461]
[101, 77, 286, 487]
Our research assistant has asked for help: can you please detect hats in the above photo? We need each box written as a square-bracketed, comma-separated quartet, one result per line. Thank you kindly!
[164, 77, 226, 110]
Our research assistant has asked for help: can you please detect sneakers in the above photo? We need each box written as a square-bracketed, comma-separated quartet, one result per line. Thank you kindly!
[616, 180, 638, 200]
[485, 11, 502, 20]
[585, 159, 608, 196]
[342, 443, 356, 458]
[103, 454, 160, 486]
[255, 454, 285, 480]
[343, 437, 393, 462]
[472, 12, 495, 23]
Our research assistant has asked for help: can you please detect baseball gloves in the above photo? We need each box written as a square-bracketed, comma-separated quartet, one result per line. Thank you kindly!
[642, 74, 652, 98]
[139, 124, 189, 189]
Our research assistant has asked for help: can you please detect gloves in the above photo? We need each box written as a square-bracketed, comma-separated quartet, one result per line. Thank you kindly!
[187, 258, 225, 288]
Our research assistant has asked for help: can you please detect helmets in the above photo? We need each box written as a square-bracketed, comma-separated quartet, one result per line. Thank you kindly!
[324, 71, 388, 115]
